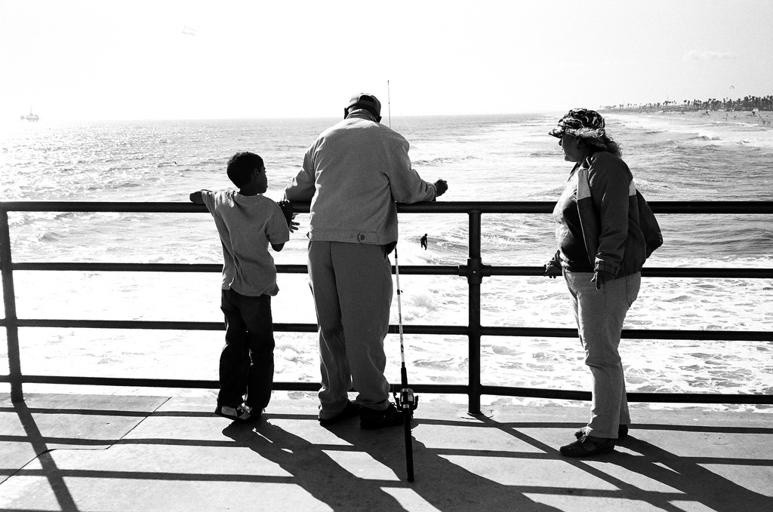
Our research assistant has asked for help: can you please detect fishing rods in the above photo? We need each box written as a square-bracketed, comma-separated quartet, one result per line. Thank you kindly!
[387, 78, 419, 484]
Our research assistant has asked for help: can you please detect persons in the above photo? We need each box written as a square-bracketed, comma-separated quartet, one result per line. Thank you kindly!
[281, 92, 448, 430]
[188, 152, 290, 427]
[420, 234, 427, 250]
[543, 107, 664, 458]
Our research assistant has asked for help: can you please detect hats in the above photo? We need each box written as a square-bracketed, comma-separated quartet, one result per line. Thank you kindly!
[346, 93, 381, 114]
[548, 108, 606, 138]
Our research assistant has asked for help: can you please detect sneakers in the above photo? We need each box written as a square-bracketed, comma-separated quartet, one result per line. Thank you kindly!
[560, 436, 615, 457]
[245, 402, 262, 416]
[319, 399, 360, 426]
[360, 403, 412, 430]
[215, 405, 251, 421]
[575, 424, 628, 439]
